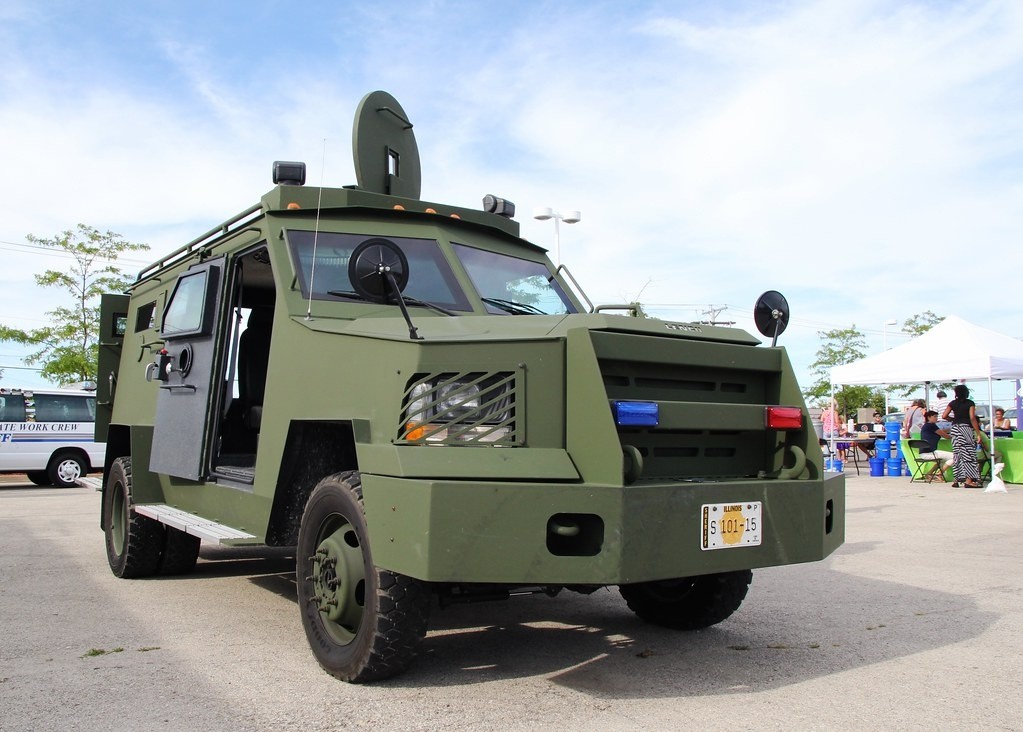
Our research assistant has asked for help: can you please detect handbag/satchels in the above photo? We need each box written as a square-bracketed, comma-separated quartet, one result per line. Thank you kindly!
[906, 426, 911, 438]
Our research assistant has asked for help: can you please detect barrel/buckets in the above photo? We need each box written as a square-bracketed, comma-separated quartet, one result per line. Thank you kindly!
[875, 440, 891, 458]
[906, 463, 912, 476]
[895, 440, 904, 458]
[884, 422, 901, 440]
[868, 458, 885, 476]
[887, 458, 901, 476]
[827, 459, 842, 472]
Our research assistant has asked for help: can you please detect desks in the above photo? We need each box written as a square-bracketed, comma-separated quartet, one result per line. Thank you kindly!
[900, 437, 1023, 485]
[846, 431, 886, 459]
[825, 438, 875, 475]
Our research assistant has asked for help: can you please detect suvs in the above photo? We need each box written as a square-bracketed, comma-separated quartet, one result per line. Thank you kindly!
[85, 86, 848, 684]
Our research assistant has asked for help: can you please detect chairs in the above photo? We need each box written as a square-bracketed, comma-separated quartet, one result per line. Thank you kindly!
[816, 438, 836, 461]
[908, 440, 947, 484]
[975, 440, 997, 483]
[237, 305, 274, 454]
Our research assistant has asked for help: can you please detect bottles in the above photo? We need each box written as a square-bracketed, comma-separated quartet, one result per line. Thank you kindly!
[976, 443, 982, 452]
[981, 423, 984, 431]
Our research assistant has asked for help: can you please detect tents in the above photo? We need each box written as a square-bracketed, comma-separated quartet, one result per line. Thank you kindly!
[828, 312, 1023, 479]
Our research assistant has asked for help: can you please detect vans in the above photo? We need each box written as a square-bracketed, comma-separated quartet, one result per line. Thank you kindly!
[0, 379, 108, 488]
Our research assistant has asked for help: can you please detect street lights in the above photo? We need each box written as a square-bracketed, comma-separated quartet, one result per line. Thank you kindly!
[533, 207, 582, 266]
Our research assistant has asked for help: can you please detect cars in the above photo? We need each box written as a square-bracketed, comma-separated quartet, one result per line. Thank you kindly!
[880, 412, 906, 423]
[1002, 406, 1023, 432]
[974, 404, 1004, 425]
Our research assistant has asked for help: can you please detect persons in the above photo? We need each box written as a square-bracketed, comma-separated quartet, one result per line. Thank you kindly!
[903, 400, 919, 434]
[819, 399, 843, 460]
[857, 410, 885, 460]
[933, 391, 954, 434]
[837, 414, 848, 463]
[918, 411, 954, 481]
[972, 415, 1003, 482]
[906, 399, 928, 437]
[942, 385, 983, 489]
[985, 407, 1011, 430]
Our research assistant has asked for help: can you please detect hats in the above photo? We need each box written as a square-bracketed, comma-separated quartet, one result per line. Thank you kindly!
[873, 412, 879, 417]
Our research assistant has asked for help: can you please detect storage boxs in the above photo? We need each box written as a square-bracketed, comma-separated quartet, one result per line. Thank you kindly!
[856, 423, 872, 431]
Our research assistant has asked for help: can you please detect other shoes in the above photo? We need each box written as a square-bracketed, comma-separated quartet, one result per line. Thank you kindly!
[964, 483, 982, 488]
[925, 474, 933, 482]
[935, 475, 946, 483]
[952, 483, 959, 487]
